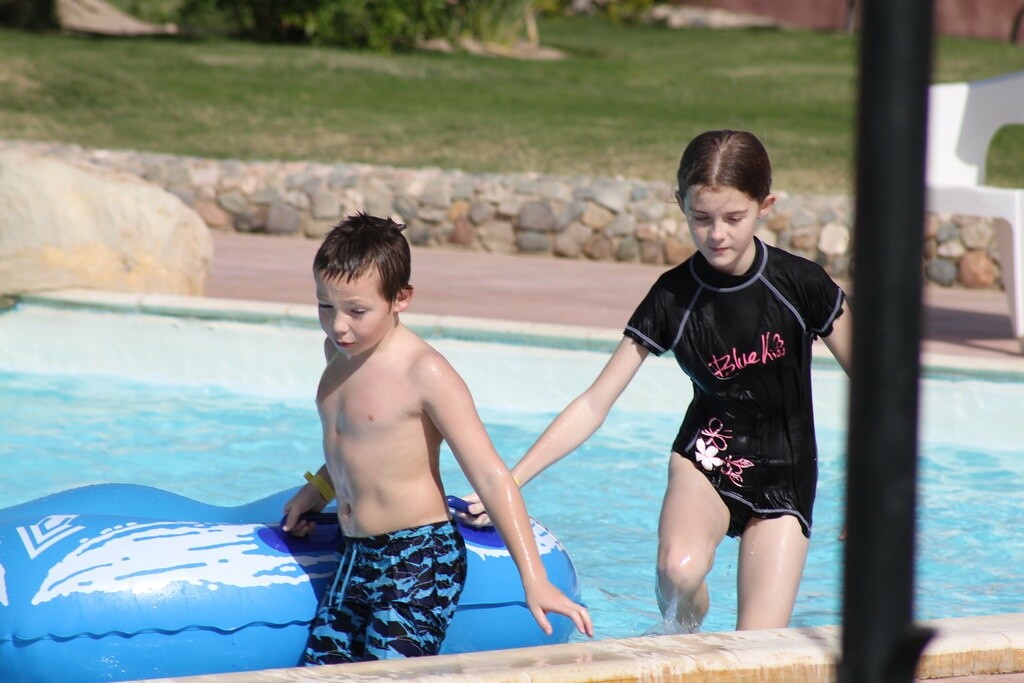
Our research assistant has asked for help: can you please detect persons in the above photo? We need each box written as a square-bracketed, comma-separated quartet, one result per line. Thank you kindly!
[455, 129, 855, 636]
[280, 210, 596, 669]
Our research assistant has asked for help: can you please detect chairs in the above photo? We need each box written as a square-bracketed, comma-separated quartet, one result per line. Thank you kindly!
[924, 68, 1024, 355]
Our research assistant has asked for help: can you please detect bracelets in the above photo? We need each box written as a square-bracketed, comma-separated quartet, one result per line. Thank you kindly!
[304, 469, 334, 505]
